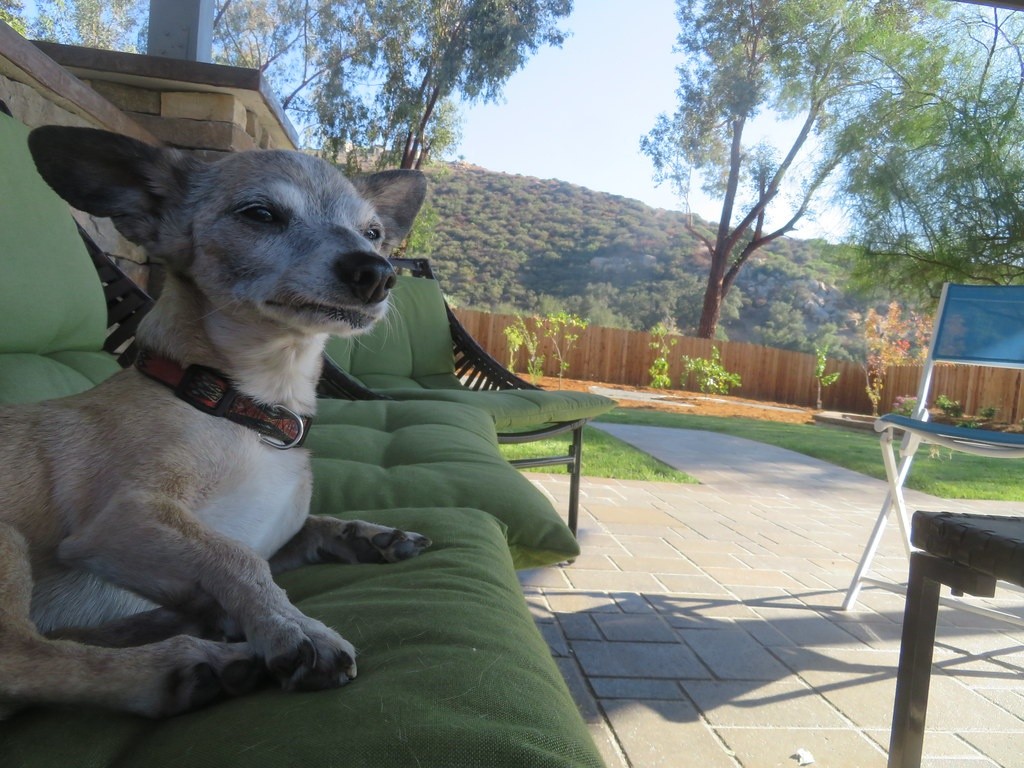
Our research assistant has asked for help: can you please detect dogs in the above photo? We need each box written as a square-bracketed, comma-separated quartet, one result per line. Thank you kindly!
[0, 123, 436, 730]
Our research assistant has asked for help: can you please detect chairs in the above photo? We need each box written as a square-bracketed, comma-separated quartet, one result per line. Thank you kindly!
[0, 503, 612, 767]
[316, 253, 619, 541]
[0, 109, 580, 572]
[839, 283, 1022, 608]
